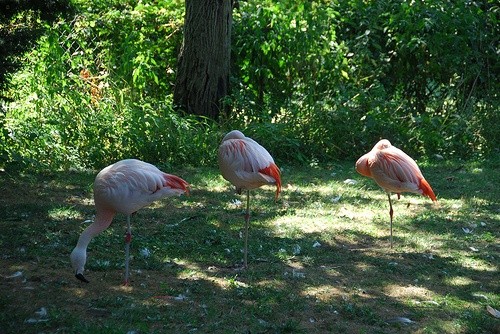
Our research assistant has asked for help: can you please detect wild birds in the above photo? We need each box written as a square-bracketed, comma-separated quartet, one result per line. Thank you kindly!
[218, 129, 281, 269]
[70, 159, 192, 284]
[356, 139, 437, 250]
